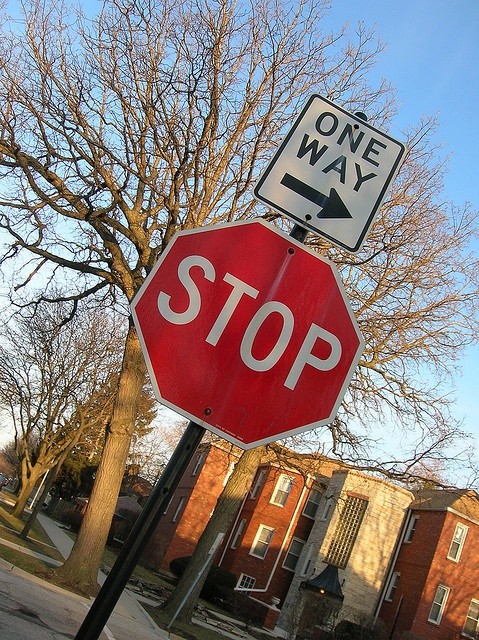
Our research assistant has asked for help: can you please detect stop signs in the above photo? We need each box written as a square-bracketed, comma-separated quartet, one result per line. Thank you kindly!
[129, 217, 364, 451]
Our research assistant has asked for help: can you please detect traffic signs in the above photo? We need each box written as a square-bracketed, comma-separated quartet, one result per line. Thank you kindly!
[252, 94, 406, 255]
